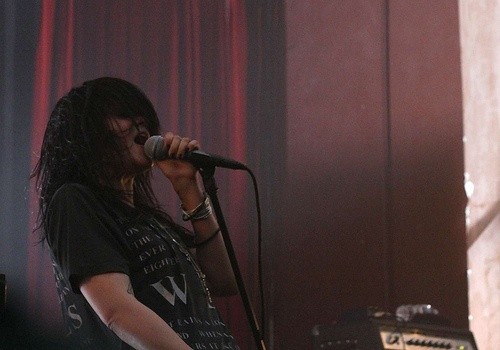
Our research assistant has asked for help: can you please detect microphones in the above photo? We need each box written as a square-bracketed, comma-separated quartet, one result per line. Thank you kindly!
[144, 136, 247, 170]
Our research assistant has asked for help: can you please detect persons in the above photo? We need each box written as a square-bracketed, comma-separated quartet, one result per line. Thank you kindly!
[29, 77, 239, 350]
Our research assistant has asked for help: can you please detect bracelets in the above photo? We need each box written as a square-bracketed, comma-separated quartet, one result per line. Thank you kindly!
[181, 192, 212, 223]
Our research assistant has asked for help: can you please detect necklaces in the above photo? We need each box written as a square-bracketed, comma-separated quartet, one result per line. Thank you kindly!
[152, 215, 214, 303]
